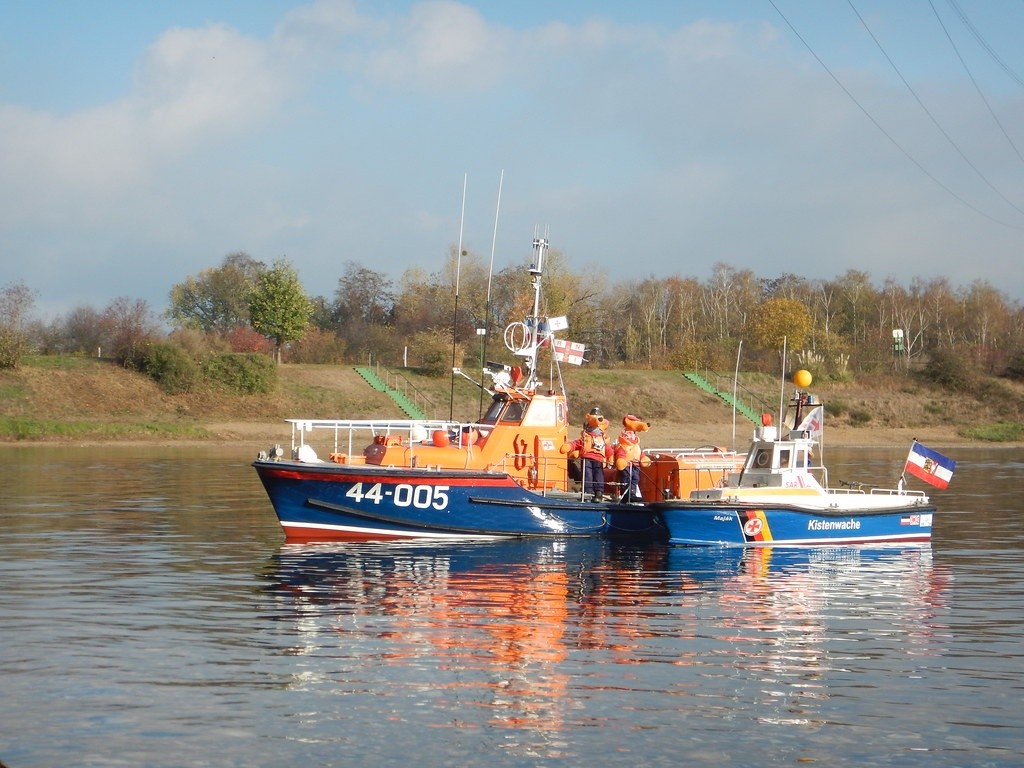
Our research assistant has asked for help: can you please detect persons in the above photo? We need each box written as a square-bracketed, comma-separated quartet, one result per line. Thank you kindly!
[615, 415, 651, 503]
[560, 408, 614, 502]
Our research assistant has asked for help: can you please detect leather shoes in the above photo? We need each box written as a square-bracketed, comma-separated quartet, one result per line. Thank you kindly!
[586, 491, 603, 503]
[623, 494, 643, 502]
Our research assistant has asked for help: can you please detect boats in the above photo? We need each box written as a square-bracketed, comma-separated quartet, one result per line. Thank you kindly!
[251, 235, 650, 539]
[650, 392, 935, 551]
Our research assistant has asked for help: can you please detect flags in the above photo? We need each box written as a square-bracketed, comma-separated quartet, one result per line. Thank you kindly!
[796, 406, 823, 438]
[548, 316, 568, 331]
[552, 339, 585, 366]
[525, 320, 550, 350]
[905, 442, 957, 490]
